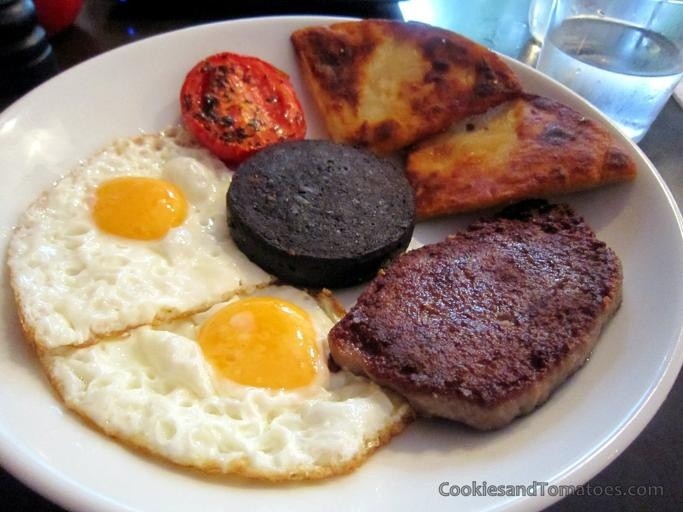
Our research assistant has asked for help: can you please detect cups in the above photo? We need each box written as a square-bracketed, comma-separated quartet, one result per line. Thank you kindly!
[535, 0, 683, 144]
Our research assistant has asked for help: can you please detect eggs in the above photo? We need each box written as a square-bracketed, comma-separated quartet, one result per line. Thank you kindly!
[4, 121, 417, 486]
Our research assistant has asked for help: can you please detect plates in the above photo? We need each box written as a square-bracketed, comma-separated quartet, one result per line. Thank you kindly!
[0, 14, 683, 512]
